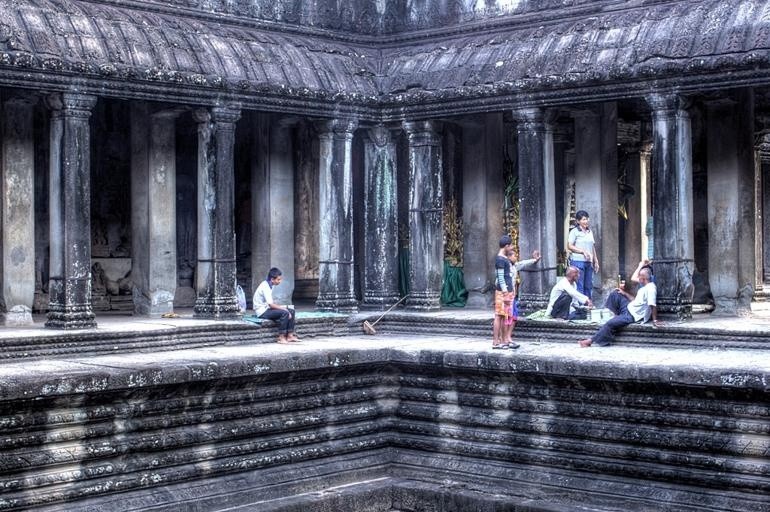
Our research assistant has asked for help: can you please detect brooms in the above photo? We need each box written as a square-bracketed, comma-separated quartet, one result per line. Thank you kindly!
[363, 290, 409, 335]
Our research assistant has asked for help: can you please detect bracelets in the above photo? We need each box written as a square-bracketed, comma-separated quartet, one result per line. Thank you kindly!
[652, 319, 658, 322]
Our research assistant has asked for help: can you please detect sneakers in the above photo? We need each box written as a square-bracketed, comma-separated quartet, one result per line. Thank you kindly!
[492, 341, 520, 350]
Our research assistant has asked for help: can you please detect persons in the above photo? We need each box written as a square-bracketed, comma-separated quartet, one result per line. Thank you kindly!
[631, 261, 656, 296]
[492, 236, 513, 350]
[566, 210, 600, 309]
[253, 267, 303, 343]
[546, 266, 594, 322]
[507, 249, 542, 348]
[578, 267, 663, 347]
